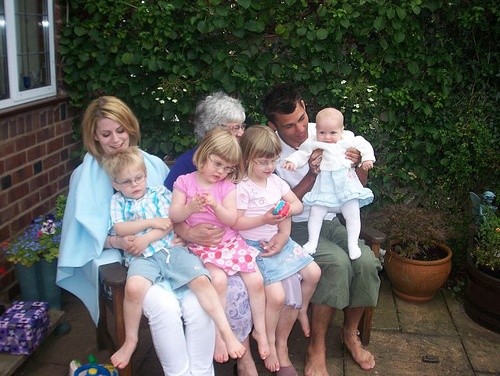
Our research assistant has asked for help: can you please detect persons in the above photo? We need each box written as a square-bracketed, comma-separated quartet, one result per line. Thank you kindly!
[262, 82, 383, 376]
[68, 96, 217, 376]
[170, 128, 271, 364]
[281, 108, 376, 261]
[102, 146, 246, 369]
[230, 125, 322, 371]
[164, 93, 302, 376]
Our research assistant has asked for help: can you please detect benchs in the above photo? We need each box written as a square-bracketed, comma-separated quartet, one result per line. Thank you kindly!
[94, 161, 387, 376]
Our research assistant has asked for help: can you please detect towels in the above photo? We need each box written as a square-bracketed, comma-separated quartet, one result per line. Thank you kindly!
[54, 146, 170, 335]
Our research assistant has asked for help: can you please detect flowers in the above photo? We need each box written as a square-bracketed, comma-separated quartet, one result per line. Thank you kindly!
[3, 212, 63, 267]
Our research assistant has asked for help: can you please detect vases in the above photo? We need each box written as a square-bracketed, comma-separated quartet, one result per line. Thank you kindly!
[10, 259, 63, 309]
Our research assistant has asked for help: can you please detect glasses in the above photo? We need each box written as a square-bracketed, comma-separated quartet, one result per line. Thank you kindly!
[207, 154, 235, 174]
[251, 155, 281, 165]
[220, 123, 247, 131]
[114, 174, 147, 187]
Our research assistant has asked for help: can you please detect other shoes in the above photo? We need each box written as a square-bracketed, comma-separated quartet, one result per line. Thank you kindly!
[277, 363, 297, 376]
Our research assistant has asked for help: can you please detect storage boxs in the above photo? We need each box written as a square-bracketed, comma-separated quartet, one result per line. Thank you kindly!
[0, 300, 49, 356]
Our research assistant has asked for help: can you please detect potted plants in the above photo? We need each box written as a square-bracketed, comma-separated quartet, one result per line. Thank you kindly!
[465, 192, 500, 335]
[367, 201, 452, 302]
